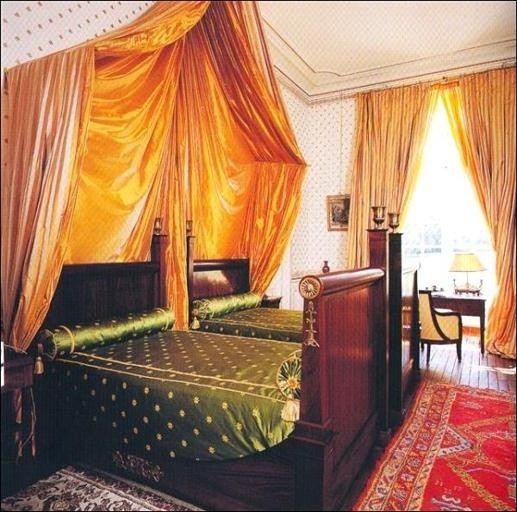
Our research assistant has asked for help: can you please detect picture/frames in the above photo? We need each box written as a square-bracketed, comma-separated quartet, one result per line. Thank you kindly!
[328, 194, 356, 231]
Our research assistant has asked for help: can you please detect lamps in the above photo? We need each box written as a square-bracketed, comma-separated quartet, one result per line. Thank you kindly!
[445, 251, 490, 296]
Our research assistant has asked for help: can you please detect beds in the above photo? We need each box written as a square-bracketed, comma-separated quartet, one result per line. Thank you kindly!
[15, 204, 440, 511]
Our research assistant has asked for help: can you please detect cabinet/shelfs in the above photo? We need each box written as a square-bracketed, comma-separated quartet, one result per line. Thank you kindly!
[433, 287, 495, 357]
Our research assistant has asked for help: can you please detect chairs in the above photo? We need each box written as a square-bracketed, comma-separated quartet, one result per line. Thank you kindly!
[418, 289, 464, 363]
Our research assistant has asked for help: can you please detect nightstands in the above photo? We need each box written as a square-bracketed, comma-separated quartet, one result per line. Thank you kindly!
[262, 295, 283, 308]
[2, 341, 68, 491]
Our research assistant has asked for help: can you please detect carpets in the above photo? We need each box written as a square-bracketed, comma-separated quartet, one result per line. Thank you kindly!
[350, 375, 516, 512]
[0, 460, 207, 511]
